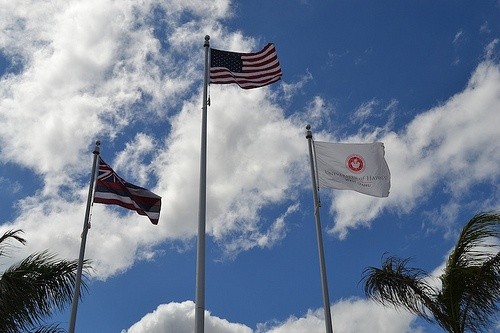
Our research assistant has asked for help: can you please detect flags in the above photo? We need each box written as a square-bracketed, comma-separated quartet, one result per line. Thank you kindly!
[313, 141, 390, 197]
[93, 156, 162, 224]
[210, 42, 282, 89]
